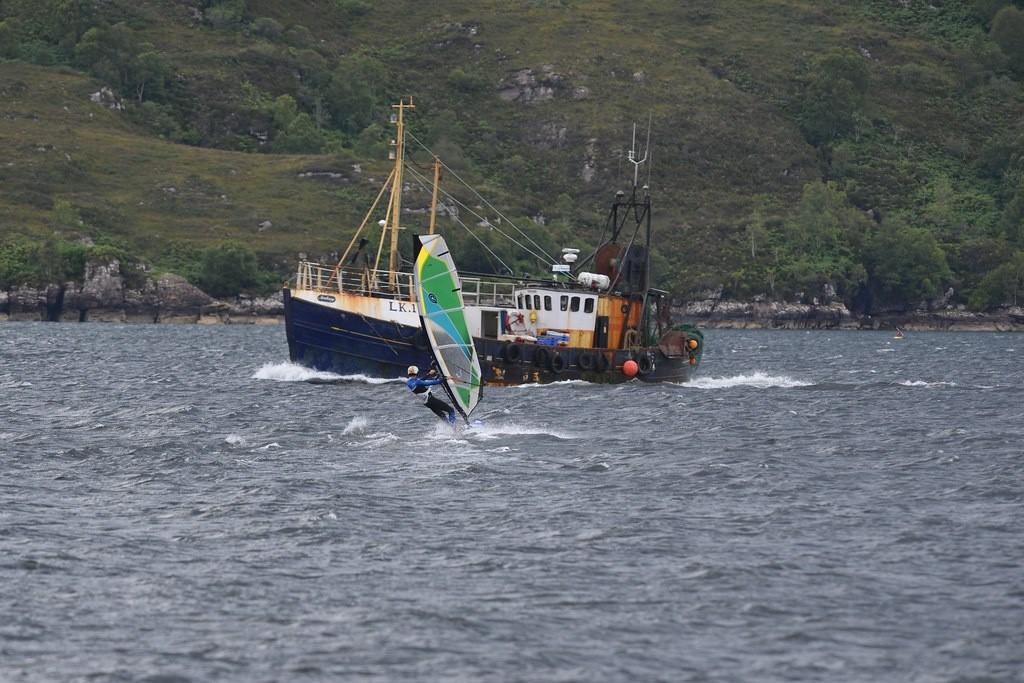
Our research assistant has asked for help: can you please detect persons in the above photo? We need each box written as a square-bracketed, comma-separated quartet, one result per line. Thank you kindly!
[406, 366, 455, 421]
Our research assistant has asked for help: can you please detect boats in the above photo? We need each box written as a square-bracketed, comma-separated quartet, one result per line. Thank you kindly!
[283, 97, 703, 387]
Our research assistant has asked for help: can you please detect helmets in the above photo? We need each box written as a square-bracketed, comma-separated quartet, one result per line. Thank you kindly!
[408, 366, 419, 374]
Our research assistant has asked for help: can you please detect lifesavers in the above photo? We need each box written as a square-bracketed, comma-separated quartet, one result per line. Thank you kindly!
[506, 313, 524, 331]
[580, 351, 592, 368]
[595, 354, 609, 372]
[414, 328, 428, 350]
[635, 355, 653, 373]
[505, 344, 520, 362]
[552, 354, 566, 373]
[536, 347, 552, 367]
